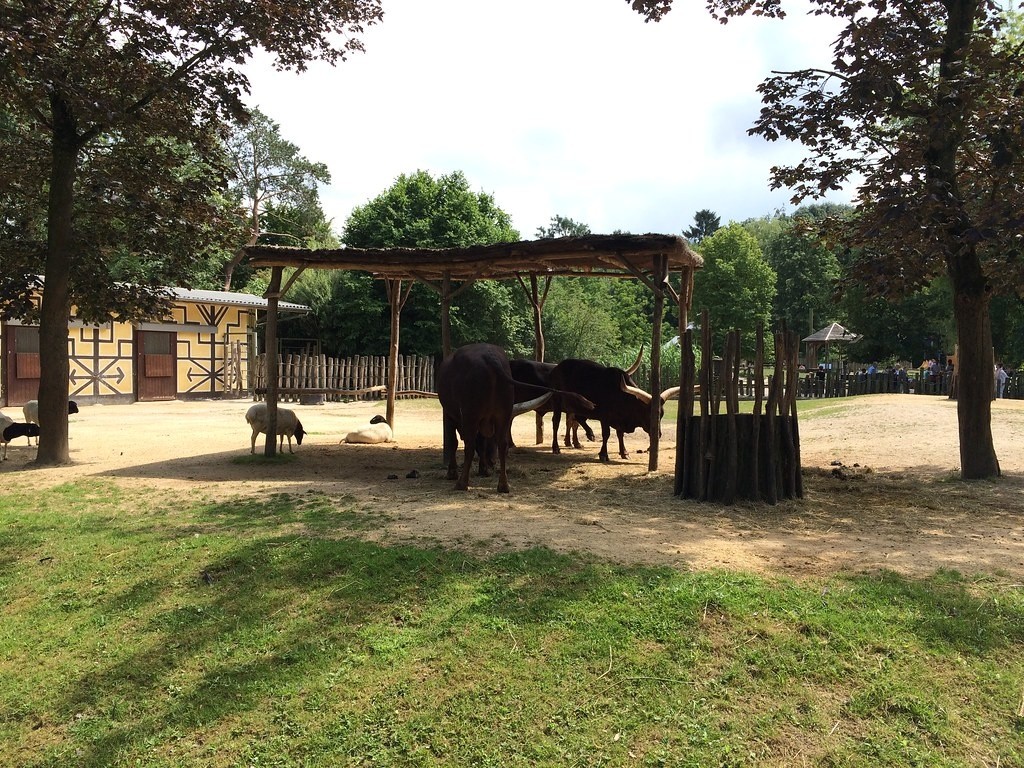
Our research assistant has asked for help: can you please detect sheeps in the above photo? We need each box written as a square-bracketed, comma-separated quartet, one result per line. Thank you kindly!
[339, 415, 398, 445]
[245, 405, 306, 456]
[0, 400, 79, 459]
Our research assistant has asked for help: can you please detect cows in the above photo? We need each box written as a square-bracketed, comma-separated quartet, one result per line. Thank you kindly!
[436, 344, 703, 494]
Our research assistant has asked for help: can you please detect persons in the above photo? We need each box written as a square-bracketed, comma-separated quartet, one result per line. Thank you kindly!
[815, 366, 826, 394]
[859, 368, 867, 385]
[798, 364, 805, 370]
[945, 359, 954, 372]
[891, 363, 914, 383]
[994, 363, 1009, 399]
[868, 362, 879, 374]
[924, 359, 941, 380]
[800, 372, 813, 387]
